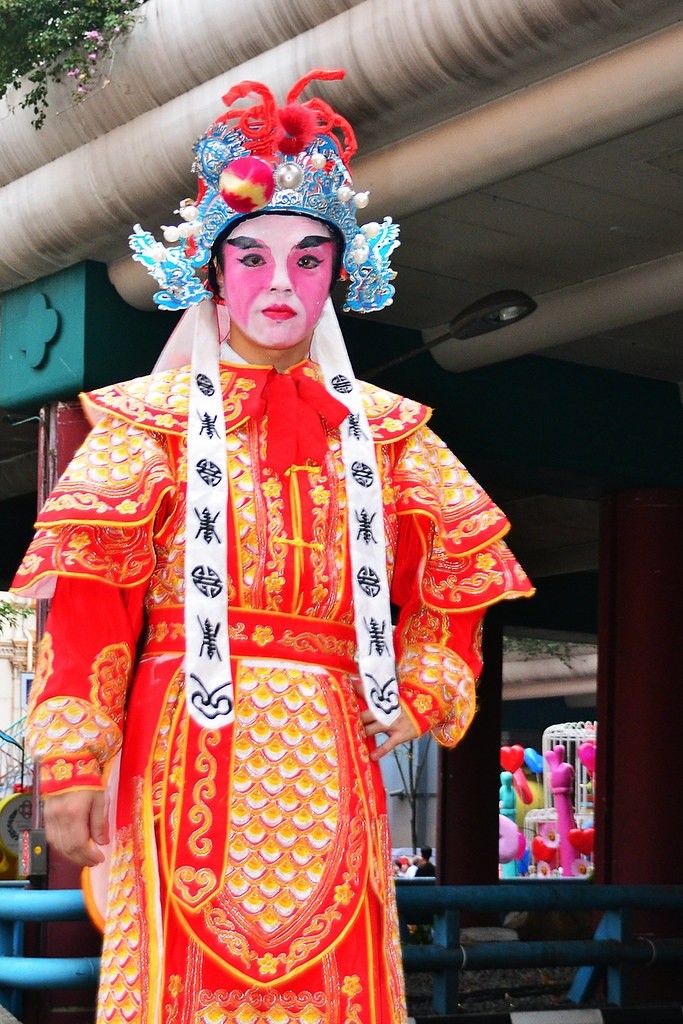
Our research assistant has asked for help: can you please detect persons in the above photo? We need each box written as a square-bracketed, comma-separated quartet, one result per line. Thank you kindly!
[392, 854, 423, 878]
[416, 845, 436, 877]
[11, 121, 535, 1024]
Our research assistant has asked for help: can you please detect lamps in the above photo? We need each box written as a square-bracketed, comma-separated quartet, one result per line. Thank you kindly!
[357, 288, 538, 382]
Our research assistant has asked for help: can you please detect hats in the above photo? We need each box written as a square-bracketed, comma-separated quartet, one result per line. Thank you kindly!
[128, 70, 403, 728]
